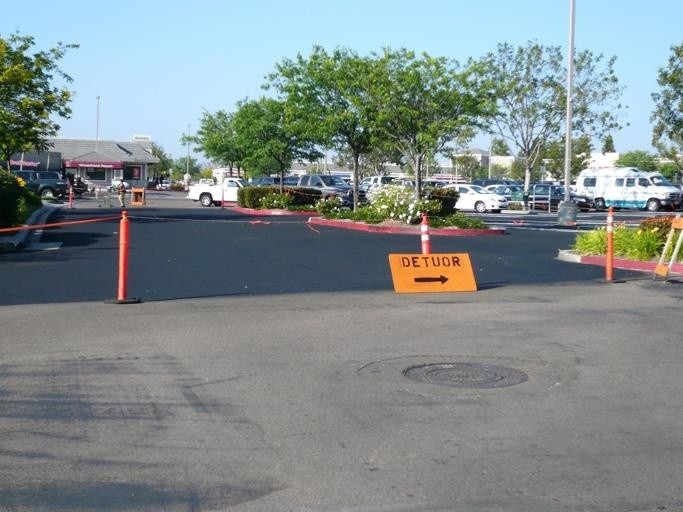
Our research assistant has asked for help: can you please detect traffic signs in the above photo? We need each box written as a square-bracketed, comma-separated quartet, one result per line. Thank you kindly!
[388, 253, 477, 293]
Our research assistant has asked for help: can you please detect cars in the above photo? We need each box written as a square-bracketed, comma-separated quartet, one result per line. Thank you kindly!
[13, 171, 66, 196]
[156, 180, 170, 190]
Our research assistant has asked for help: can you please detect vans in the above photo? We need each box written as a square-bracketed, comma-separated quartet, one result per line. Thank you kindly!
[576, 167, 682, 211]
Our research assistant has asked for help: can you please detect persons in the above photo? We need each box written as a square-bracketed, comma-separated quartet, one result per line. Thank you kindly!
[118, 180, 126, 207]
[151, 174, 167, 191]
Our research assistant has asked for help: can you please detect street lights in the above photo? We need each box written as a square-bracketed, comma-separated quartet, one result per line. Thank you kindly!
[96, 95, 100, 139]
[186, 124, 190, 173]
